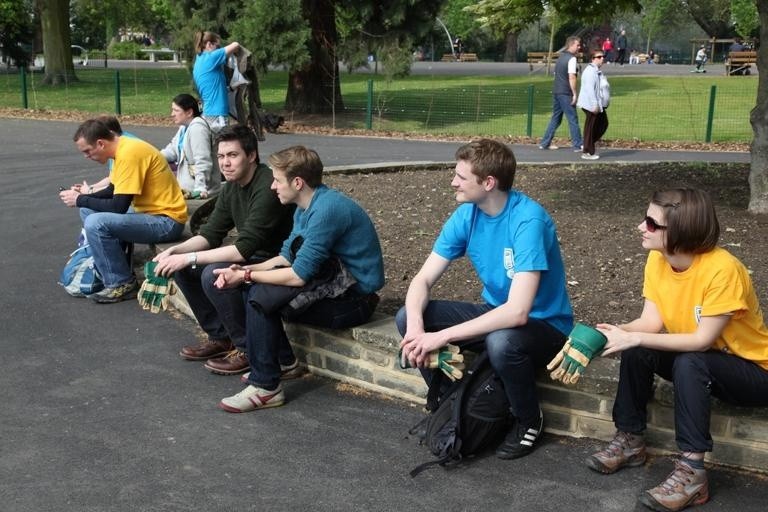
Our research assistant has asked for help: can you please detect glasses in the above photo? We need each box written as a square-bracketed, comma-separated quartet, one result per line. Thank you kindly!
[643, 209, 671, 233]
[591, 54, 607, 59]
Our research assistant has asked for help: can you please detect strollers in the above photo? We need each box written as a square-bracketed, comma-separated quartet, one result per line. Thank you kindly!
[730, 43, 753, 75]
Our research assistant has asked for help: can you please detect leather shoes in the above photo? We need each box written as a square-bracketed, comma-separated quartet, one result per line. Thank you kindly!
[177, 337, 237, 362]
[202, 352, 255, 376]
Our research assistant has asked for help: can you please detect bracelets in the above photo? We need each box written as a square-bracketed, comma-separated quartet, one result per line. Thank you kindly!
[190, 251, 197, 270]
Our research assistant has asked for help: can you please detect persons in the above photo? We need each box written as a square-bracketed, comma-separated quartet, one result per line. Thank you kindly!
[613, 28, 627, 67]
[537, 35, 584, 152]
[583, 186, 766, 512]
[193, 30, 242, 138]
[395, 138, 574, 462]
[727, 36, 751, 75]
[628, 48, 640, 64]
[693, 43, 705, 72]
[645, 49, 654, 64]
[601, 34, 613, 65]
[574, 47, 610, 159]
[152, 125, 298, 375]
[452, 37, 463, 61]
[158, 93, 221, 199]
[60, 113, 188, 303]
[211, 146, 386, 413]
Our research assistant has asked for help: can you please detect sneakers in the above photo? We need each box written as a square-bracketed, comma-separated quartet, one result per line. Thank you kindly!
[495, 405, 545, 460]
[241, 358, 305, 385]
[539, 144, 559, 150]
[85, 278, 141, 305]
[571, 145, 583, 152]
[581, 152, 600, 160]
[219, 382, 286, 414]
[582, 430, 648, 476]
[637, 458, 710, 512]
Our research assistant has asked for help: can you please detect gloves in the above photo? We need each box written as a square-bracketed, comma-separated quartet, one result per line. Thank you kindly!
[135, 259, 178, 316]
[396, 339, 467, 385]
[544, 318, 610, 385]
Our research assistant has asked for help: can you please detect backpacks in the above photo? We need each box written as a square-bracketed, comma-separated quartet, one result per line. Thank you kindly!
[422, 350, 514, 461]
[56, 227, 107, 298]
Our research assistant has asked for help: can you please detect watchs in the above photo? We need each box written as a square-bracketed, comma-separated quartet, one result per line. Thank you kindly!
[244, 269, 255, 286]
